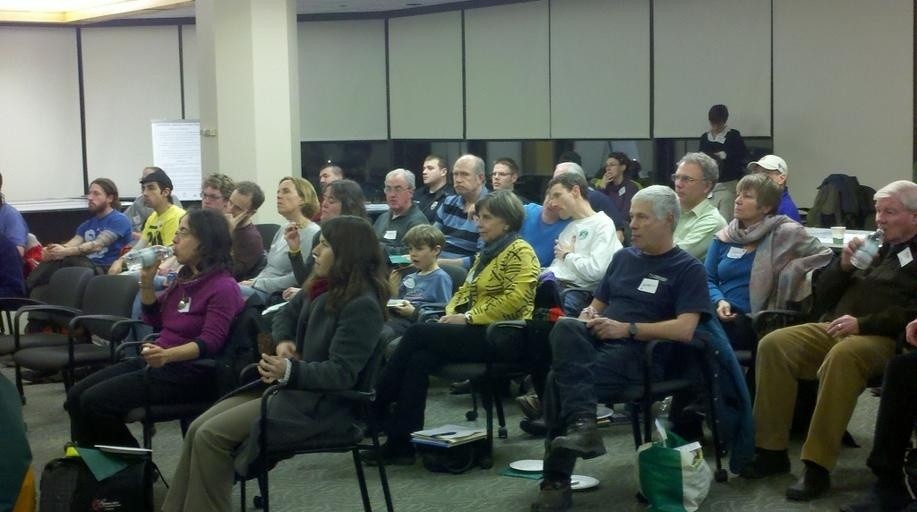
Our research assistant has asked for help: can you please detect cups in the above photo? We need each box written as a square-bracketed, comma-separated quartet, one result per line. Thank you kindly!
[830, 226, 846, 246]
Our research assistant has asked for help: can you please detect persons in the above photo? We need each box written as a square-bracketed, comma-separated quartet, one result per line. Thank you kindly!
[0, 103, 917, 511]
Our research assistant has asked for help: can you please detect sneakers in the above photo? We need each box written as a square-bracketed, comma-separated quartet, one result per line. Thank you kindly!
[517, 394, 547, 436]
[360, 435, 416, 466]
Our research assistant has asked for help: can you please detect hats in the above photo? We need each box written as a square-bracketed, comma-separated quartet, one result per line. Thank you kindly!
[749, 154, 788, 178]
[140, 172, 173, 189]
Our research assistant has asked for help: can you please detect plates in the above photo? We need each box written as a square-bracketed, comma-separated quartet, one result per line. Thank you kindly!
[507, 457, 545, 472]
[568, 474, 598, 490]
[93, 444, 155, 456]
[595, 405, 614, 419]
[386, 298, 410, 308]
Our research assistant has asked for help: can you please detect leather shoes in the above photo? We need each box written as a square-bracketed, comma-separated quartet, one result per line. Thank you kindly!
[786, 464, 830, 500]
[738, 448, 790, 477]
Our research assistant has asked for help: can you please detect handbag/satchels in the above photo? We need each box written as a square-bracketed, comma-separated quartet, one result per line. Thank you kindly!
[422, 440, 490, 472]
[38, 455, 155, 512]
[636, 418, 712, 512]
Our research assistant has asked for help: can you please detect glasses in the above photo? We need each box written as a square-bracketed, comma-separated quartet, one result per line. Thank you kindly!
[201, 193, 224, 200]
[671, 174, 711, 184]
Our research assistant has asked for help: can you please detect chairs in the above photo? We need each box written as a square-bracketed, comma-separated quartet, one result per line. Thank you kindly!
[732, 252, 861, 450]
[103, 311, 245, 510]
[1, 265, 98, 406]
[253, 224, 281, 251]
[394, 225, 593, 471]
[13, 275, 137, 411]
[238, 333, 394, 509]
[601, 328, 728, 503]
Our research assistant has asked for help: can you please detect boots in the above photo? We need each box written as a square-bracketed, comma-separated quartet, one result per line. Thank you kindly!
[531, 479, 572, 512]
[551, 412, 606, 458]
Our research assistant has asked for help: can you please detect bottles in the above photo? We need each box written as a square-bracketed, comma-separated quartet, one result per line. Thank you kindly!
[848, 227, 884, 270]
[120, 244, 174, 272]
